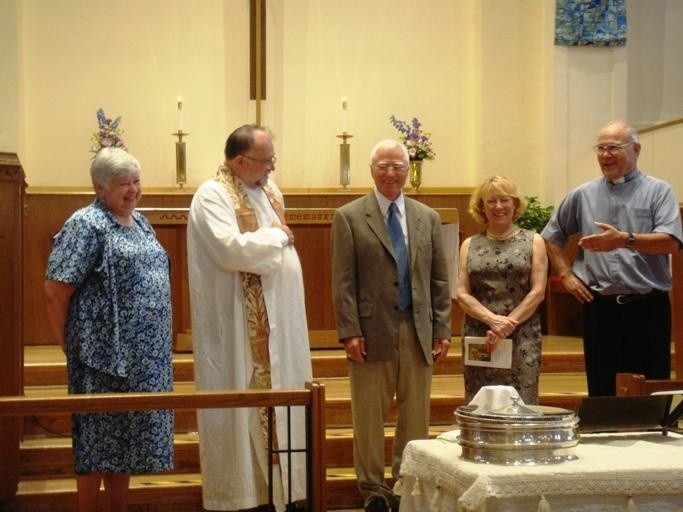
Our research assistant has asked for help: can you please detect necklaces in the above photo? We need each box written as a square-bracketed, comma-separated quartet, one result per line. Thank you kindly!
[485, 225, 517, 238]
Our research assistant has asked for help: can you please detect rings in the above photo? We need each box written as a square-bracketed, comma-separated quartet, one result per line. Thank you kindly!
[348, 354, 351, 357]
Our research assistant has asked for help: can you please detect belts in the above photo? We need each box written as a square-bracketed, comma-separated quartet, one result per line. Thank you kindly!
[598, 294, 657, 304]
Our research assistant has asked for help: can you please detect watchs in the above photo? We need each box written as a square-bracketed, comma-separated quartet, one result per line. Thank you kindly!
[624, 231, 635, 249]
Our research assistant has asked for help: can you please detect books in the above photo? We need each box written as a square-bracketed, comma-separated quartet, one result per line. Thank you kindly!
[461, 334, 514, 371]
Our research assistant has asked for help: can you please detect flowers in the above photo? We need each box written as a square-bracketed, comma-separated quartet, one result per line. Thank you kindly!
[88, 106, 127, 163]
[388, 114, 436, 163]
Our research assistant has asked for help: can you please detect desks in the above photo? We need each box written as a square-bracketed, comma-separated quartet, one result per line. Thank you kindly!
[398, 431, 683, 511]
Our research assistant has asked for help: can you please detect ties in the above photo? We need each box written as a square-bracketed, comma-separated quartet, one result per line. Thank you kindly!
[388, 203, 409, 311]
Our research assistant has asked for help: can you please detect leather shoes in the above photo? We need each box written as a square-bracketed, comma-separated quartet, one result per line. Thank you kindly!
[364, 498, 389, 511]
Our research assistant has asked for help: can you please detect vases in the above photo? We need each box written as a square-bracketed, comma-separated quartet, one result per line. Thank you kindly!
[408, 158, 422, 193]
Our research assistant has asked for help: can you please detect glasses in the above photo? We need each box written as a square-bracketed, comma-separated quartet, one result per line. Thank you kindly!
[593, 144, 632, 154]
[243, 154, 275, 165]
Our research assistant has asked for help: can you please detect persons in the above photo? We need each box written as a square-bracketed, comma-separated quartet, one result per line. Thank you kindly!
[453, 172, 550, 410]
[543, 119, 682, 398]
[186, 122, 316, 512]
[327, 138, 454, 512]
[41, 146, 176, 511]
